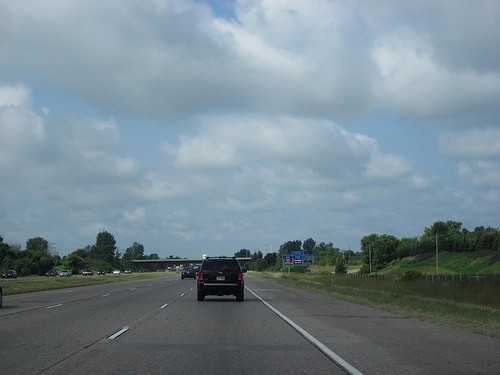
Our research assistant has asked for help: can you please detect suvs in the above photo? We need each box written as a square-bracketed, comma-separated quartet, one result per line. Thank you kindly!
[196, 256, 248, 302]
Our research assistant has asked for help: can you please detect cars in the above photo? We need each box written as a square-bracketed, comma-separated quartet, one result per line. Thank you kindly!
[180, 267, 197, 280]
[97, 269, 105, 275]
[112, 269, 121, 274]
[125, 269, 132, 274]
[192, 264, 202, 276]
[82, 269, 94, 276]
[45, 270, 57, 277]
[60, 270, 73, 278]
[2, 270, 18, 279]
